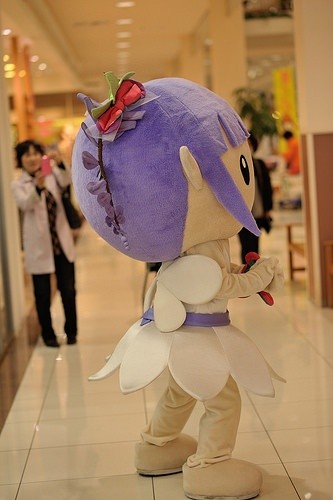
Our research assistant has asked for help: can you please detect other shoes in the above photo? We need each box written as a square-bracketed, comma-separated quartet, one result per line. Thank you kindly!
[42, 332, 59, 347]
[67, 333, 78, 344]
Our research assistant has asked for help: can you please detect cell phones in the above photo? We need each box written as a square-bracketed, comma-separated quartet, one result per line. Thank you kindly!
[41, 155, 53, 176]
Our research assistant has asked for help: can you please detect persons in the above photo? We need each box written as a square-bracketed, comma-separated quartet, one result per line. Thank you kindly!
[280, 128, 303, 177]
[9, 140, 84, 348]
[71, 70, 281, 500]
[237, 131, 273, 272]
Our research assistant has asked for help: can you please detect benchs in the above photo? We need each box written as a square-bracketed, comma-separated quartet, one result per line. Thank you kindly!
[287, 223, 305, 280]
